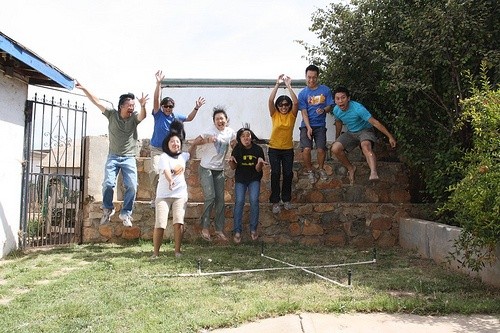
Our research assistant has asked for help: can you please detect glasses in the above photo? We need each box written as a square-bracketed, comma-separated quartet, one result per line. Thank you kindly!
[278, 102, 290, 107]
[162, 105, 174, 108]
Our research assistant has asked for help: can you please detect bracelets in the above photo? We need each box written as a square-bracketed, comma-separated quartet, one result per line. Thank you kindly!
[194, 107, 198, 111]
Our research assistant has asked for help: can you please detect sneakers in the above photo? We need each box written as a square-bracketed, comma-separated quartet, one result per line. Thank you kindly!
[118, 212, 133, 227]
[99, 208, 115, 225]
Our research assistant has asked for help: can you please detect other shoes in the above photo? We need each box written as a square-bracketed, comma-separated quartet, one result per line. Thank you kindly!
[279, 199, 291, 210]
[234, 233, 242, 244]
[200, 230, 212, 242]
[215, 231, 227, 241]
[317, 167, 329, 181]
[250, 231, 258, 241]
[307, 170, 317, 184]
[272, 203, 280, 214]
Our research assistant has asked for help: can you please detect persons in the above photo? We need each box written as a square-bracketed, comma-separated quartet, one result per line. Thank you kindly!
[192, 105, 238, 243]
[298, 65, 333, 185]
[149, 120, 188, 262]
[74, 78, 150, 228]
[149, 68, 206, 184]
[267, 72, 298, 215]
[331, 87, 397, 185]
[225, 122, 270, 245]
